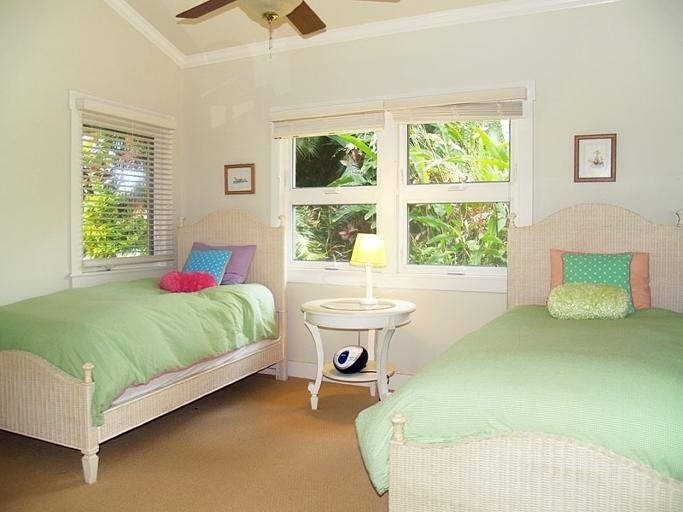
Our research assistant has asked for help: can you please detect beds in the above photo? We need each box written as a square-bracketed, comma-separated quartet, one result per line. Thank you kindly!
[389, 204, 683, 512]
[0, 209, 290, 484]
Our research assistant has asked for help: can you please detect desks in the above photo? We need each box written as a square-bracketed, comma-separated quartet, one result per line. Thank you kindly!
[301, 299, 417, 410]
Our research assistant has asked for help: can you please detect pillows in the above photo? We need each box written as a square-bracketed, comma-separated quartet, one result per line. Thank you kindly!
[548, 249, 652, 321]
[160, 241, 258, 293]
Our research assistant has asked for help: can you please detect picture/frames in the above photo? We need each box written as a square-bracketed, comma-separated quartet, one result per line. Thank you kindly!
[225, 163, 255, 195]
[574, 133, 616, 182]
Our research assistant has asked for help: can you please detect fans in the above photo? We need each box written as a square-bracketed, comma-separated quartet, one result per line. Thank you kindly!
[176, 0, 403, 35]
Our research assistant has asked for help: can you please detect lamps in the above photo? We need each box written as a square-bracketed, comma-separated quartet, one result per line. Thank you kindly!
[237, 0, 305, 20]
[350, 233, 386, 305]
